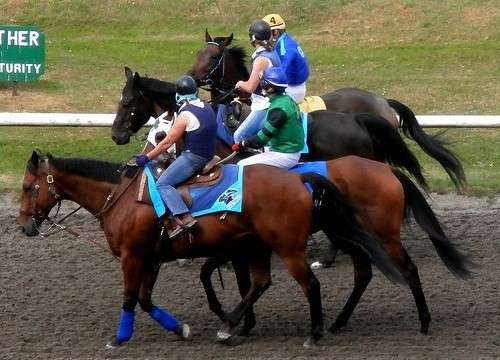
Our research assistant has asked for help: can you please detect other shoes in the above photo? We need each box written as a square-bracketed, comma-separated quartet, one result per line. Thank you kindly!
[169, 214, 197, 239]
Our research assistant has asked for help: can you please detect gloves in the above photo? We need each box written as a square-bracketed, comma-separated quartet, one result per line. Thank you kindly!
[136, 154, 149, 167]
[232, 144, 240, 151]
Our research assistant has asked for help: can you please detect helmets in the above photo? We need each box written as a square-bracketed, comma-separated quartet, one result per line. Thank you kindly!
[176, 76, 197, 102]
[262, 14, 285, 30]
[259, 67, 288, 92]
[249, 20, 271, 40]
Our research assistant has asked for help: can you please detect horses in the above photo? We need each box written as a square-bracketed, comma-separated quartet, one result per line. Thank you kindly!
[20, 148, 407, 348]
[110, 66, 434, 269]
[186, 26, 468, 195]
[141, 106, 476, 340]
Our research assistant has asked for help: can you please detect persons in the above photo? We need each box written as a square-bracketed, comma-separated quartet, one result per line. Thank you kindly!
[232, 19, 287, 143]
[262, 13, 308, 104]
[232, 67, 305, 174]
[135, 76, 218, 241]
